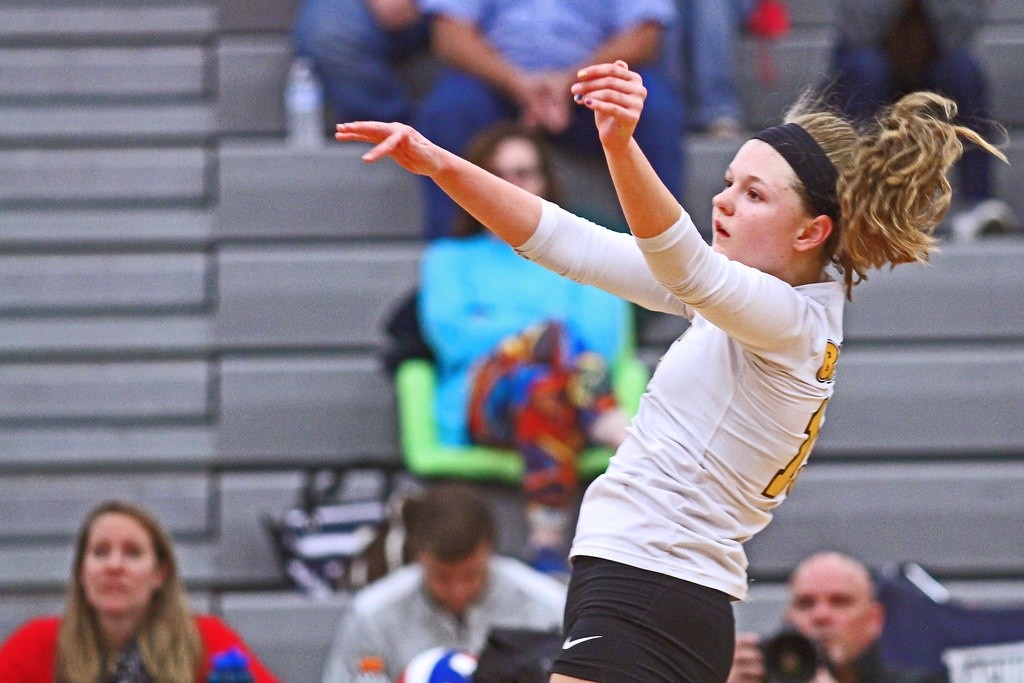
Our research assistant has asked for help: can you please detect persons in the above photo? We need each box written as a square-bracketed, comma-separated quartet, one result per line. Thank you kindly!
[287, 0, 1016, 242]
[730, 549, 915, 683]
[0, 499, 289, 683]
[388, 116, 656, 582]
[319, 491, 568, 682]
[335, 59, 1010, 682]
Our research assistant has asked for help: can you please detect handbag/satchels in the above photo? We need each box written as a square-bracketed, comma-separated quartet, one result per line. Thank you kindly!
[277, 458, 408, 595]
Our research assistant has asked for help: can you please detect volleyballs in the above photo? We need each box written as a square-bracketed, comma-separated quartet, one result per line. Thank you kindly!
[394, 645, 478, 683]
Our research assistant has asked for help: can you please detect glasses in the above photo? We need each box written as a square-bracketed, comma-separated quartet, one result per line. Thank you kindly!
[493, 167, 545, 183]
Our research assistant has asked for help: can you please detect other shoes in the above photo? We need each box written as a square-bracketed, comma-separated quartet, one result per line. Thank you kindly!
[969, 201, 1010, 238]
[711, 115, 742, 141]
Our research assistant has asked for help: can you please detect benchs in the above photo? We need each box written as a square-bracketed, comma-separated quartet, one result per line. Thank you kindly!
[218, 0, 1024, 591]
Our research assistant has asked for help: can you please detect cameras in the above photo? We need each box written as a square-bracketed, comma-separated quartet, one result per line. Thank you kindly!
[750, 622, 830, 683]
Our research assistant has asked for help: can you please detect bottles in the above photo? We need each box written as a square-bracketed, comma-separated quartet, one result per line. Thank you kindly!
[286, 57, 324, 148]
[207, 646, 255, 683]
[356, 658, 394, 683]
